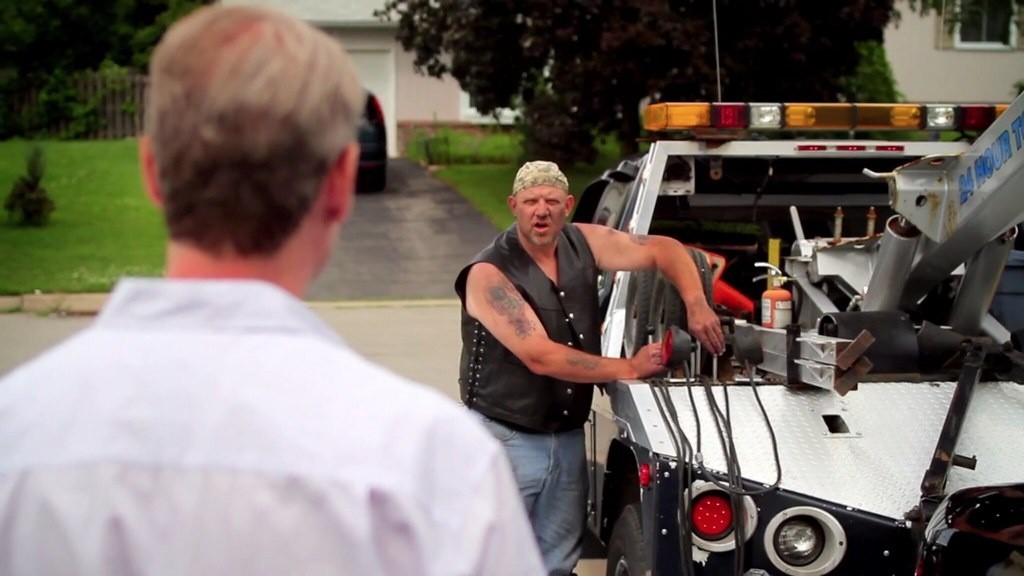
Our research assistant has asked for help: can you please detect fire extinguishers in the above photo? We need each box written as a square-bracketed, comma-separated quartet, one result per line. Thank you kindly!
[752, 261, 801, 330]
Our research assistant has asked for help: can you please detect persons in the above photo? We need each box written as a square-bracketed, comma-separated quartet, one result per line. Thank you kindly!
[455, 162, 726, 576]
[0, 6, 548, 576]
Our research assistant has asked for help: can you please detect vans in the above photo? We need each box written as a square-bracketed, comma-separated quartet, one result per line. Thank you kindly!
[355, 92, 388, 193]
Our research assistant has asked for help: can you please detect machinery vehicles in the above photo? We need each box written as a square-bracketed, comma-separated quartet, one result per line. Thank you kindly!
[570, 88, 1024, 576]
